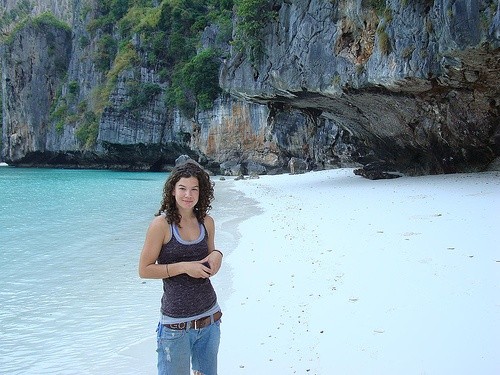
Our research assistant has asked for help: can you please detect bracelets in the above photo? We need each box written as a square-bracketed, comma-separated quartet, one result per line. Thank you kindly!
[166, 264, 170, 278]
[211, 249, 223, 257]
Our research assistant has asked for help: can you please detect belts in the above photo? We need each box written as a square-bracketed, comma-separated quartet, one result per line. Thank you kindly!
[164, 311, 222, 330]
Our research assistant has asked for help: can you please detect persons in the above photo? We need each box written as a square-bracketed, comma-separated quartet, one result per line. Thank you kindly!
[139, 163, 223, 375]
